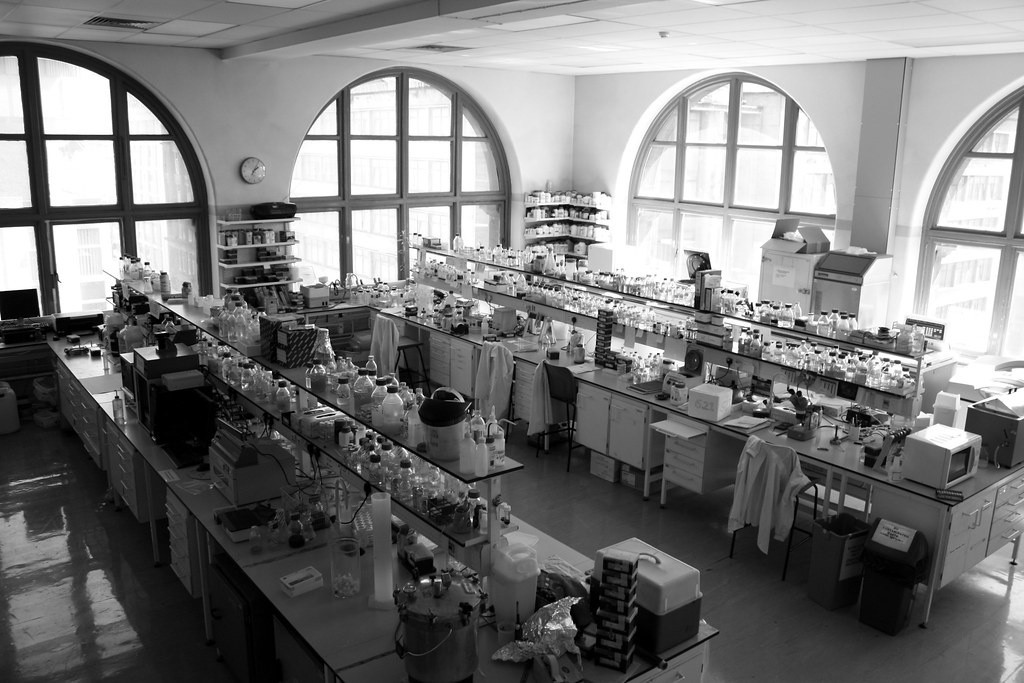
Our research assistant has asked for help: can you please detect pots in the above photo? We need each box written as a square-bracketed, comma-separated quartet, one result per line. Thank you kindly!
[394, 564, 488, 683]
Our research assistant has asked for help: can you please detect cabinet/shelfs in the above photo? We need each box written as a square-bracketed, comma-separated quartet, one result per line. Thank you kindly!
[217, 216, 303, 291]
[97, 251, 524, 613]
[228, 272, 1024, 629]
[43, 279, 721, 683]
[411, 236, 938, 435]
[525, 188, 614, 260]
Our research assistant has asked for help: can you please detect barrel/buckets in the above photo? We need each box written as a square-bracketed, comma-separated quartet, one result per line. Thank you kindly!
[0, 381, 20, 434]
[419, 387, 471, 462]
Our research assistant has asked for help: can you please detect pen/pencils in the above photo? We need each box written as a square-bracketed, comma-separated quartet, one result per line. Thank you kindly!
[776, 429, 788, 436]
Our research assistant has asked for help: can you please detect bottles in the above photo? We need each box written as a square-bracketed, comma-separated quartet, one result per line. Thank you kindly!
[182, 281, 300, 415]
[347, 231, 486, 335]
[486, 242, 697, 405]
[120, 254, 170, 293]
[218, 228, 275, 246]
[289, 513, 306, 548]
[459, 405, 506, 477]
[351, 442, 487, 535]
[112, 390, 123, 419]
[717, 286, 925, 444]
[305, 356, 425, 444]
[524, 187, 611, 241]
[249, 526, 261, 553]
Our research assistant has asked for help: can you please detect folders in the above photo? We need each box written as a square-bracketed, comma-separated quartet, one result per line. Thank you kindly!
[626, 378, 664, 395]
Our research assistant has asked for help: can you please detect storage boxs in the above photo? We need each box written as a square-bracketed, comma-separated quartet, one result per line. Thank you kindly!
[760, 218, 830, 254]
[587, 537, 702, 656]
[274, 345, 322, 370]
[258, 313, 306, 364]
[688, 383, 733, 422]
[276, 325, 321, 351]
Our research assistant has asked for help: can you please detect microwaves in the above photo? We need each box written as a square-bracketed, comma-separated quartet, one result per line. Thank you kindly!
[902, 423, 982, 490]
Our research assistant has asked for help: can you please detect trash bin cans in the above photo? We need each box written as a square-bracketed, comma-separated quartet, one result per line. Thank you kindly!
[805, 511, 873, 613]
[855, 517, 933, 637]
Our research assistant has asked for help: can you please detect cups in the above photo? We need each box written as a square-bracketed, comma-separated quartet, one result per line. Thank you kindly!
[329, 538, 360, 598]
[498, 621, 514, 646]
